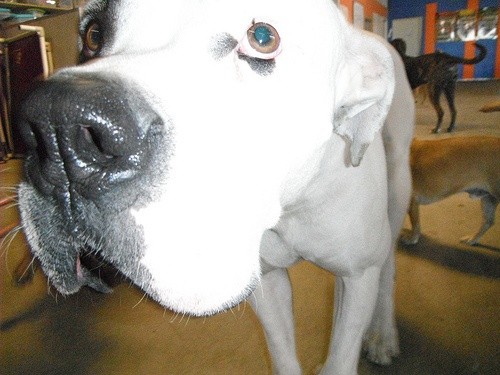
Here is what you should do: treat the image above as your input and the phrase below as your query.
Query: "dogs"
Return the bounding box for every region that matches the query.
[388,38,487,133]
[0,0,414,375]
[401,136,500,245]
